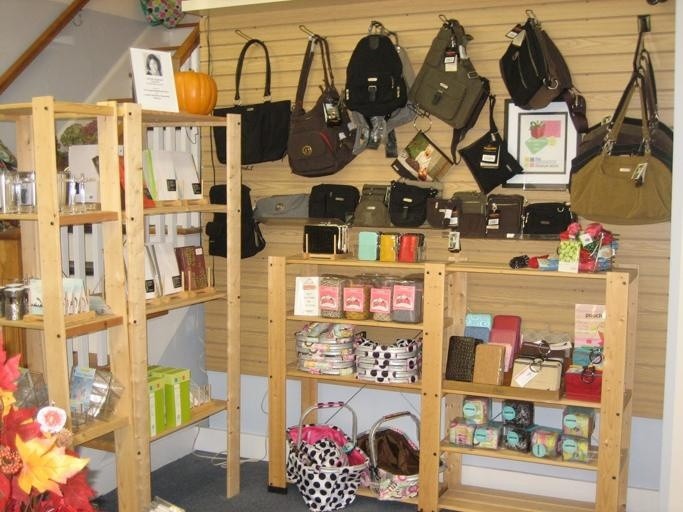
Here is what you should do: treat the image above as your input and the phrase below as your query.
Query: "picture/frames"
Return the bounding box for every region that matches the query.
[501,97,582,192]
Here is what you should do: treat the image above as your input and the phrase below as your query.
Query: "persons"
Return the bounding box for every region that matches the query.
[143,53,163,77]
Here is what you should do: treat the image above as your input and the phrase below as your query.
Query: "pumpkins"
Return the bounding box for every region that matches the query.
[175,67,218,115]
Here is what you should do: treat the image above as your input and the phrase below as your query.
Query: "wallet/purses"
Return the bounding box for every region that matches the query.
[145,241,210,301]
[465,312,492,329]
[464,325,490,344]
[493,314,521,331]
[357,231,426,262]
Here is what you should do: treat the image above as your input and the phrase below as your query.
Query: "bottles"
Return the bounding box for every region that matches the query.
[0,169,37,213]
[0,274,29,321]
[319,272,424,323]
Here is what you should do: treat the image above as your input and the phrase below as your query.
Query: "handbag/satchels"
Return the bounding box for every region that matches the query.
[206,184,266,259]
[285,35,353,178]
[213,39,291,166]
[340,21,416,144]
[489,191,524,241]
[384,181,439,227]
[301,184,360,252]
[510,356,562,392]
[406,14,492,131]
[453,191,487,238]
[565,368,602,401]
[571,345,604,366]
[457,96,524,195]
[473,345,505,384]
[445,335,483,382]
[354,183,389,227]
[389,130,454,186]
[522,341,573,362]
[499,10,590,132]
[523,201,572,238]
[490,329,519,373]
[568,50,674,226]
[428,194,459,227]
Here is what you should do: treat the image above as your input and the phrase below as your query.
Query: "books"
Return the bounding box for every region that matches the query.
[68,365,95,415]
[120,243,208,300]
[82,370,112,419]
[90,154,156,212]
[141,149,201,201]
